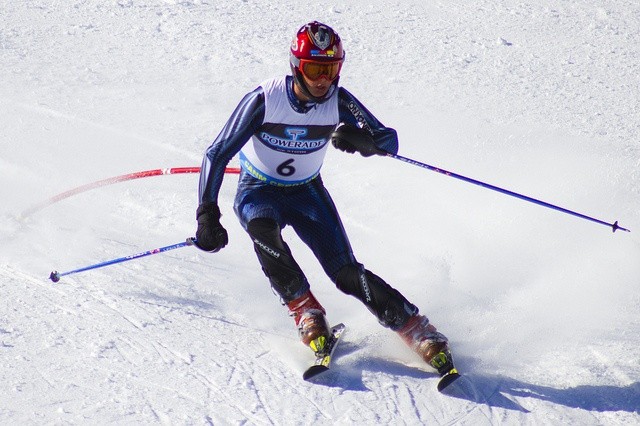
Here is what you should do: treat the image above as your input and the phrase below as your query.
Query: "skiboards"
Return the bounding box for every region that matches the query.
[301,323,461,392]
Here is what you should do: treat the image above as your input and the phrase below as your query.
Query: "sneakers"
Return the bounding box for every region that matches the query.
[396,315,449,362]
[286,290,329,346]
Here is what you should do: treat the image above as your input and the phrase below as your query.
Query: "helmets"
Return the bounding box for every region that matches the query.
[290,21,345,103]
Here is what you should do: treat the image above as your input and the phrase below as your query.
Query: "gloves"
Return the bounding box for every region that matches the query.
[195,203,228,252]
[331,124,375,157]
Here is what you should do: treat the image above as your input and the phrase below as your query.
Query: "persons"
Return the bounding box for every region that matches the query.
[192,19,450,370]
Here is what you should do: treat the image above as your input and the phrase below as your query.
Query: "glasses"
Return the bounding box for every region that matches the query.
[290,50,345,81]
